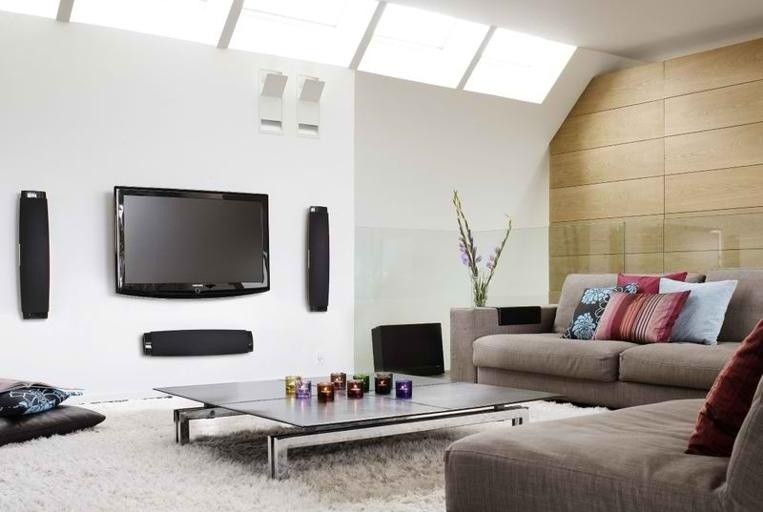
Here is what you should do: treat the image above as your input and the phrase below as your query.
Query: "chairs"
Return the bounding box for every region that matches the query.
[437,376,762,512]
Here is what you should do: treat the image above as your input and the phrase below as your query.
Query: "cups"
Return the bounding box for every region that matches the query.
[285,372,413,401]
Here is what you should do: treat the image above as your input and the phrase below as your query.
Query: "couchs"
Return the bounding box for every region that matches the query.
[447,268,762,410]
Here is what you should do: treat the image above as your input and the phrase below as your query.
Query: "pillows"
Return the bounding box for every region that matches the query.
[614,268,689,293]
[0,403,107,446]
[589,286,690,345]
[0,386,86,420]
[556,280,641,340]
[680,314,761,456]
[656,275,739,348]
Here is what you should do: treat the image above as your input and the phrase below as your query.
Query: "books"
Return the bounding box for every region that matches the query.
[0,378,63,393]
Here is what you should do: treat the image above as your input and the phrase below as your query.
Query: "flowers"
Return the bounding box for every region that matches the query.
[450,186,513,307]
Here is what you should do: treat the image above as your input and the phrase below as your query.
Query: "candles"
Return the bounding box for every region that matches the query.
[278,369,415,403]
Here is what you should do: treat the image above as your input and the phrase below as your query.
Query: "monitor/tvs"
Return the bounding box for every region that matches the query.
[112,185,270,300]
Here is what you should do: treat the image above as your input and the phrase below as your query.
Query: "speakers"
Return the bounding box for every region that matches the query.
[142,329,254,356]
[307,206,330,312]
[18,190,49,319]
